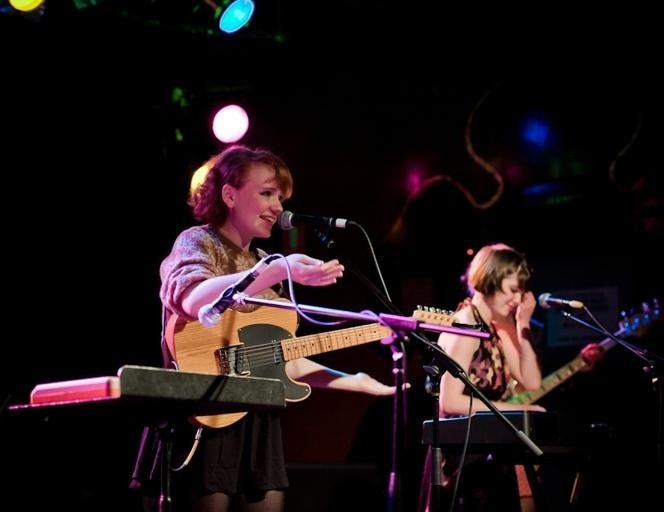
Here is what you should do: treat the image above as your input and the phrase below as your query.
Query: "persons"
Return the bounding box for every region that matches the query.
[127,143,412,510]
[418,239,547,512]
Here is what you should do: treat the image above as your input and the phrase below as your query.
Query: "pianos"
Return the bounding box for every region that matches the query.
[420,404,662,461]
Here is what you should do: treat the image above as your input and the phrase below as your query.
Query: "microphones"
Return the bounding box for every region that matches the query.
[538,293,584,310]
[197,256,272,328]
[277,210,357,231]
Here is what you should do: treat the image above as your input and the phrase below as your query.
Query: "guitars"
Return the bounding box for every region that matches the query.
[166,281,486,432]
[438,296,664,490]
